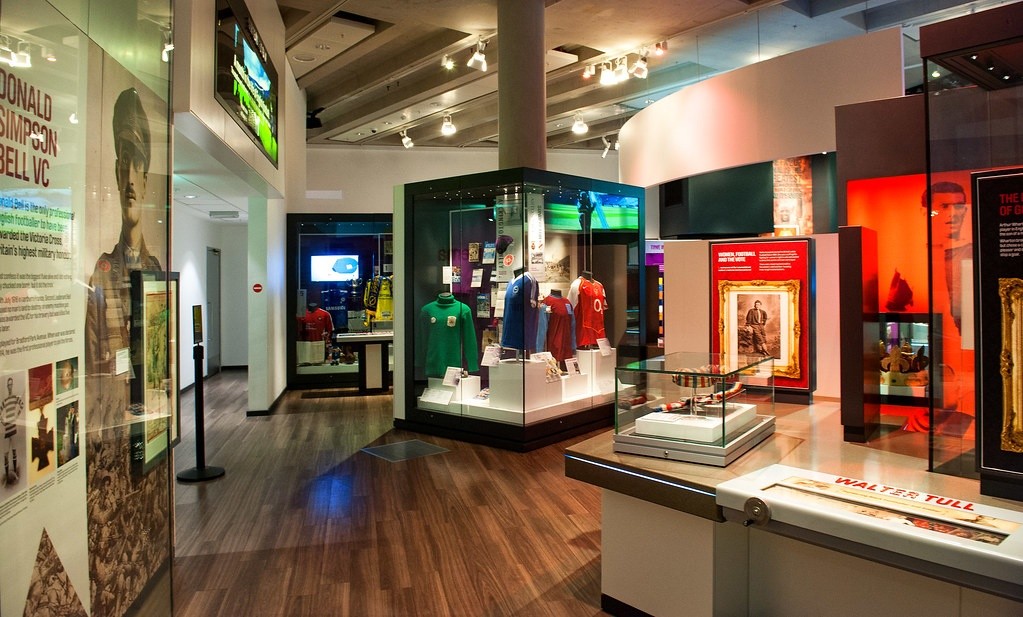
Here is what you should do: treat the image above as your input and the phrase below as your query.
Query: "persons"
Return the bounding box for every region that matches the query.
[746,300,771,355]
[501,266,538,350]
[84,86,162,435]
[0,377,24,479]
[297,303,335,358]
[885,182,973,312]
[415,292,479,378]
[566,271,608,346]
[536,289,577,361]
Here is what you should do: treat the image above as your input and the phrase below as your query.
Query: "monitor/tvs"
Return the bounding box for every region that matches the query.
[311,255,359,281]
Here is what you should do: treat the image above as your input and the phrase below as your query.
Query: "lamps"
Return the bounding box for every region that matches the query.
[572,112,588,135]
[400,130,414,149]
[584,42,664,85]
[442,114,456,135]
[467,38,490,72]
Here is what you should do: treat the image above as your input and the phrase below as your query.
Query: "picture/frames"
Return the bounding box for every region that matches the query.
[709,237,817,393]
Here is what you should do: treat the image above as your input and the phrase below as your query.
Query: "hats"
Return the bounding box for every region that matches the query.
[496,235,514,254]
[113,87,151,170]
